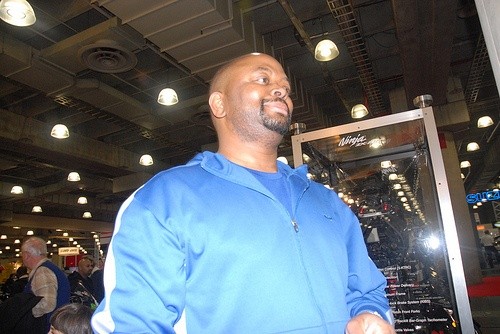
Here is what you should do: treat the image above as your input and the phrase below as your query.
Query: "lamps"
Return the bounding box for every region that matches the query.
[50,106,69,139]
[277,100,500,244]
[314,17,339,62]
[0,156,104,258]
[139,139,153,166]
[0,0,36,27]
[157,67,179,106]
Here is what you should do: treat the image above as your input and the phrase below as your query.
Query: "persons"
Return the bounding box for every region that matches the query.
[480,230,500,268]
[0,236,104,334]
[90,52,396,334]
